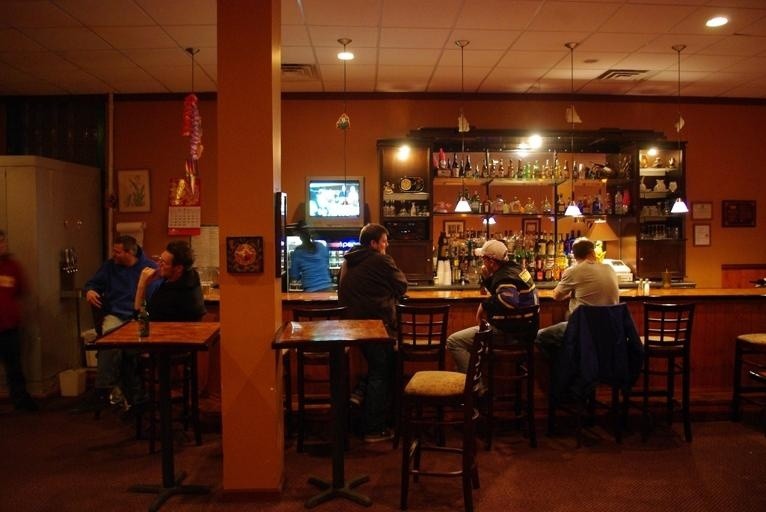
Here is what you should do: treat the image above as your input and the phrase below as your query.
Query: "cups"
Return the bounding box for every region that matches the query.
[201,284,211,297]
[661,272,673,289]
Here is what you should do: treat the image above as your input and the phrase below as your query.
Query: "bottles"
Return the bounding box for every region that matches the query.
[640,178,667,194]
[384,201,428,216]
[328,251,348,282]
[642,225,680,241]
[137,299,150,338]
[431,146,633,283]
[643,201,671,217]
[638,278,650,292]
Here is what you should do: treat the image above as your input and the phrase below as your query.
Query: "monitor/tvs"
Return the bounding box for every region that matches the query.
[305,175,365,228]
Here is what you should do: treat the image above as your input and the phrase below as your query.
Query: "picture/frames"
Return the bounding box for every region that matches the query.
[693,221,711,247]
[115,169,152,214]
[690,200,714,220]
[223,234,267,275]
[444,220,465,239]
[720,198,757,228]
[522,217,540,236]
[274,189,286,280]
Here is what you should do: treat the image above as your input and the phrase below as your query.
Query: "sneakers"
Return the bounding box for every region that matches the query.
[351,382,364,406]
[365,427,395,443]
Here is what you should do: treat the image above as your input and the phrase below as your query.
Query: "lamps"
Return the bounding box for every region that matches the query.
[561,40,583,217]
[452,39,474,215]
[483,215,496,225]
[669,43,691,217]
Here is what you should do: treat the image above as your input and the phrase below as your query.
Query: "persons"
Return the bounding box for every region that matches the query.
[0,230,34,410]
[536,240,620,362]
[309,184,360,217]
[446,239,540,400]
[106,239,207,417]
[336,222,408,443]
[288,232,334,293]
[81,235,158,405]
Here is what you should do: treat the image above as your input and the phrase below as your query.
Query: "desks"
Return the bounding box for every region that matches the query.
[83,317,221,511]
[271,317,397,512]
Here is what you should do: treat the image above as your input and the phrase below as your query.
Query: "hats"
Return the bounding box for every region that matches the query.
[474,239,509,261]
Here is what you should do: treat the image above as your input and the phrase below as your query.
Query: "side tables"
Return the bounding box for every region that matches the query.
[200,283,765,405]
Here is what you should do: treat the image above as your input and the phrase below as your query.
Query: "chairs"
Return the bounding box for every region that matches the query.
[546,300,633,446]
[286,303,453,454]
[628,301,697,444]
[401,319,498,510]
[730,333,765,430]
[74,291,201,458]
[476,303,542,451]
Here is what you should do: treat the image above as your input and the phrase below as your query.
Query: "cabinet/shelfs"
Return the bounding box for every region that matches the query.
[370,121,688,289]
[0,149,104,405]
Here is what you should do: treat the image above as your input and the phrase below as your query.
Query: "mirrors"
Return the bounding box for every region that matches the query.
[432,148,625,269]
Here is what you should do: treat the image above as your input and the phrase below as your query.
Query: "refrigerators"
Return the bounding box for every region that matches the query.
[284,225,364,291]
